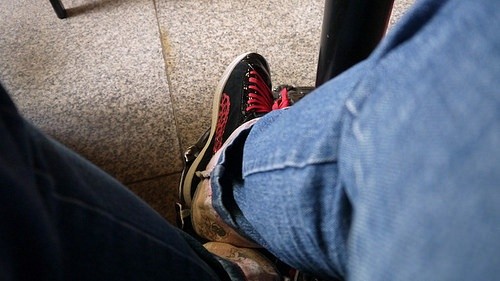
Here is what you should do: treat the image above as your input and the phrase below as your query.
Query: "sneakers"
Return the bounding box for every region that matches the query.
[183,52,290,208]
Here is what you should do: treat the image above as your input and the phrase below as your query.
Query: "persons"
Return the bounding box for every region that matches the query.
[0,0,500,281]
